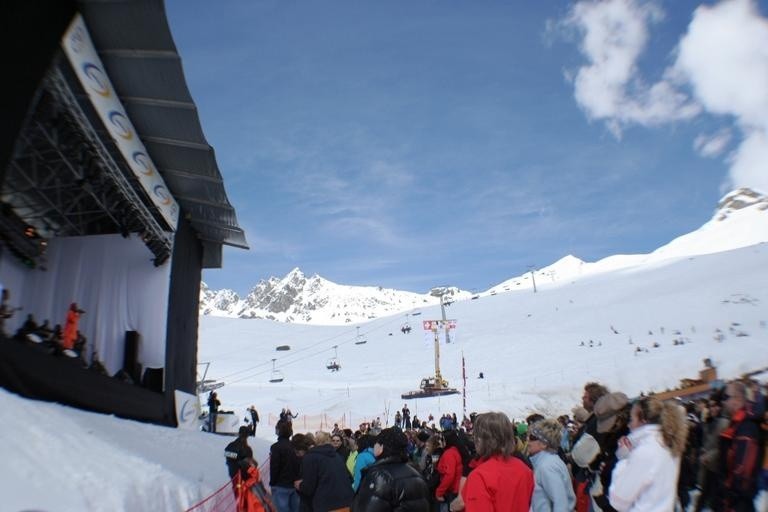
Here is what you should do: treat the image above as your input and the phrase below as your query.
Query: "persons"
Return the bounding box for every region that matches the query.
[512,380,689,512]
[1,289,15,334]
[24,302,108,376]
[225,404,535,512]
[401,325,408,333]
[676,357,768,512]
[202,390,221,434]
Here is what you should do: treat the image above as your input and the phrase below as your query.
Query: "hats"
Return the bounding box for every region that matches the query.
[594,392,629,434]
[368,428,409,449]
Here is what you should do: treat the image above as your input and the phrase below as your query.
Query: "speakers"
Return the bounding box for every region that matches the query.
[143,367,164,393]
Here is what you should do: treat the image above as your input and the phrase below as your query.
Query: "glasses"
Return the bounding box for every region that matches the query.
[529,434,539,442]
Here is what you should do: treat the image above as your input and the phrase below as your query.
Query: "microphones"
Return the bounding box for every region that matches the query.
[76,308,86,314]
